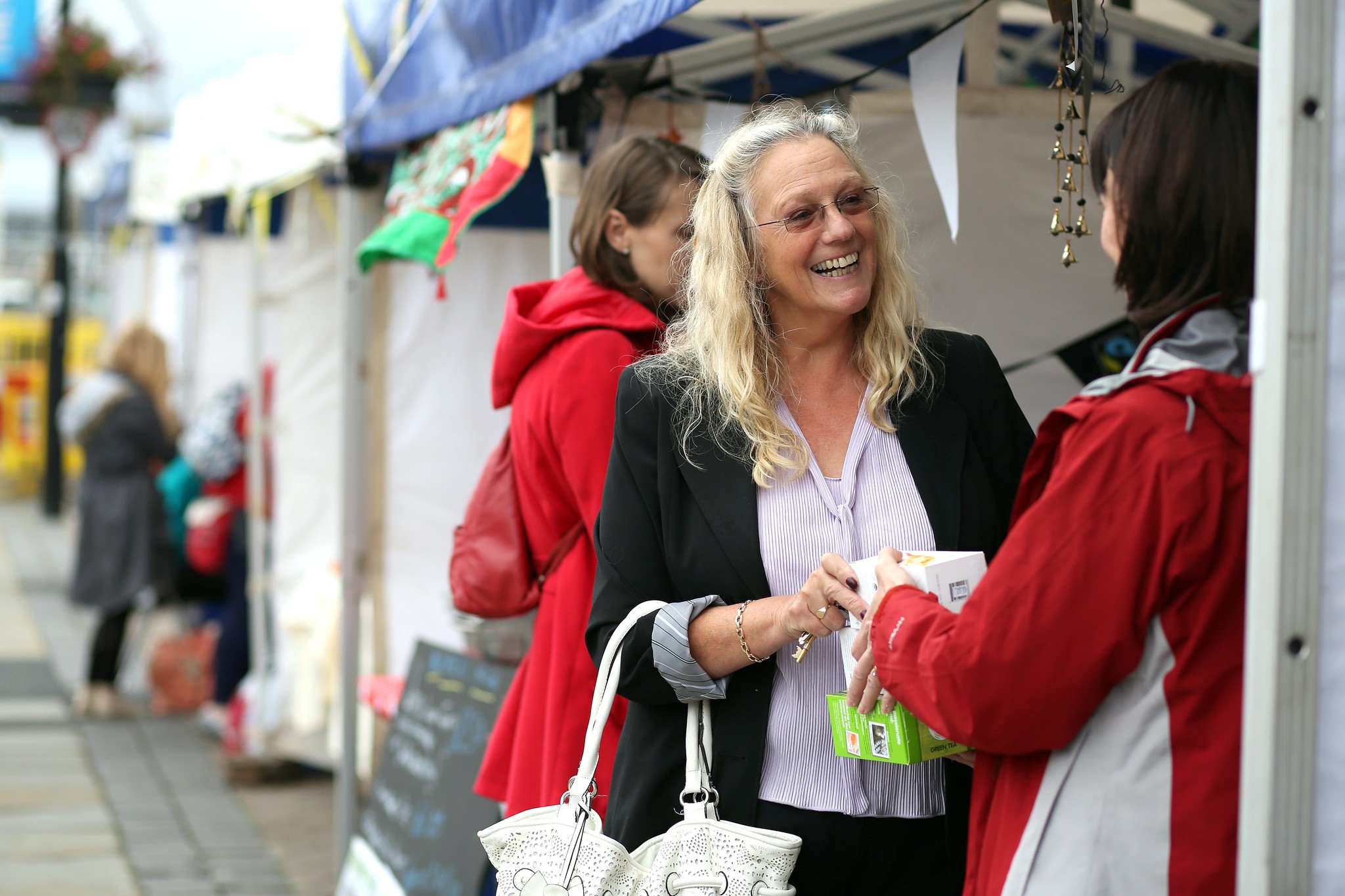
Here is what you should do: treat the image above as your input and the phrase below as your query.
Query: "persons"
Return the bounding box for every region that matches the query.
[54,326,187,717]
[193,359,276,741]
[581,97,1037,895]
[845,57,1260,896]
[472,133,716,896]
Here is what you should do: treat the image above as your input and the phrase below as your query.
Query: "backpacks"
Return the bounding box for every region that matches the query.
[450,420,587,622]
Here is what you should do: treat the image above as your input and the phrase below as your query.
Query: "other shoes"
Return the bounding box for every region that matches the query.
[72,682,236,742]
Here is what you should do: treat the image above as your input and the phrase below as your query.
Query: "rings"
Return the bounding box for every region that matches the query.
[872,664,878,677]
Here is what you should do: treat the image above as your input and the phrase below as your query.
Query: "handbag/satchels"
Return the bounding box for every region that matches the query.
[476,600,804,896]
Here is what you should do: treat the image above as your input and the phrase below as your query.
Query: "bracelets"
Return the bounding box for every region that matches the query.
[735,600,770,663]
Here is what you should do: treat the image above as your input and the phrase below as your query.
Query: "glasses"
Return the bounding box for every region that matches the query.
[744,184,879,233]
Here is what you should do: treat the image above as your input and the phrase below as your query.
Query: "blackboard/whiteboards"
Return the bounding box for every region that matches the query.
[355,640,523,894]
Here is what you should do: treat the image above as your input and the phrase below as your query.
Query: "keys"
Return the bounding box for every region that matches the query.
[791,600,849,664]
